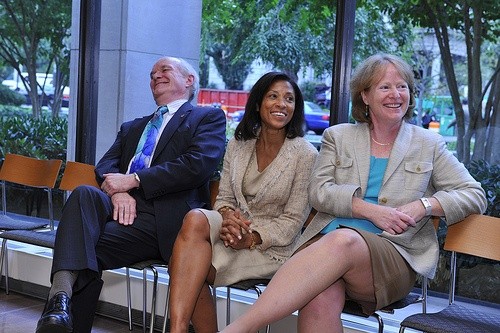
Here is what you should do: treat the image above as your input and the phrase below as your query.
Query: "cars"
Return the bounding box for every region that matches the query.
[404,95,470,137]
[10,82,70,107]
[227,100,330,135]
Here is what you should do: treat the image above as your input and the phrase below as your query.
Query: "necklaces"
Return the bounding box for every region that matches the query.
[371,136,393,146]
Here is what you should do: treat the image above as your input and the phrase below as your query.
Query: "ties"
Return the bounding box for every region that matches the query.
[129,105,169,175]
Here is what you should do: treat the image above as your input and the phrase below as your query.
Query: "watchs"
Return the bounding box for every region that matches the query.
[134,172,140,185]
[419,197,432,217]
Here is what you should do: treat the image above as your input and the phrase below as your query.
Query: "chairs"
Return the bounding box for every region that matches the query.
[0,153,98,294]
[397,213,500,333]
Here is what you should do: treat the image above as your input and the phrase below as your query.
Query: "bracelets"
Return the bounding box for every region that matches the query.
[249,233,256,250]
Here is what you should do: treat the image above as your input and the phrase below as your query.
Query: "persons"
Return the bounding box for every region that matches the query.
[216,53,488,333]
[168,71,320,333]
[34,56,226,333]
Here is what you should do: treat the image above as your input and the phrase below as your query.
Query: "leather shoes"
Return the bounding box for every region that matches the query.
[36,288,72,333]
